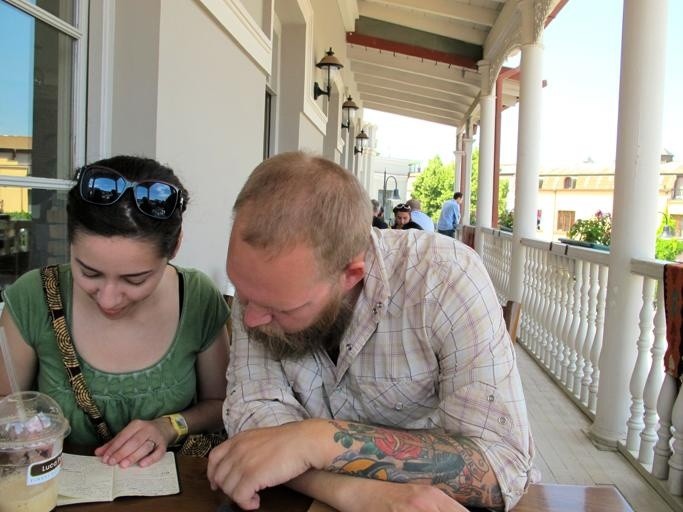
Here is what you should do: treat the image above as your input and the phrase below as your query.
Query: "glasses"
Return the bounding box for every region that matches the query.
[396,203,411,210]
[74,164,184,220]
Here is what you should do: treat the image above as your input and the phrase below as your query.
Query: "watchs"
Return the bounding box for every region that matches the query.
[162,413,189,444]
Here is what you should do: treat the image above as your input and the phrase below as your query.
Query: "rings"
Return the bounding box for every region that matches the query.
[146,438,157,449]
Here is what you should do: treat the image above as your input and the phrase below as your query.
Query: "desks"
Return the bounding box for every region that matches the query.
[0,446,636,512]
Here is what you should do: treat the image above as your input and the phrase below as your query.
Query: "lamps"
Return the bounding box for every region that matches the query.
[311,46,369,154]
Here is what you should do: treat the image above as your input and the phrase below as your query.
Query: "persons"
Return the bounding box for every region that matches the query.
[371,198,435,231]
[437,192,464,238]
[205,150,541,512]
[0,154,231,468]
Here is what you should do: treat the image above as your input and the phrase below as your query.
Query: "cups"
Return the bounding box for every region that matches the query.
[0,391,71,512]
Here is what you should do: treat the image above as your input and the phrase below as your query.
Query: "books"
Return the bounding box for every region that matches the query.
[57,451,183,506]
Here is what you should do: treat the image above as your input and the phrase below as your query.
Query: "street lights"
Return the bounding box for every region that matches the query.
[656,209,673,242]
[382,168,400,219]
